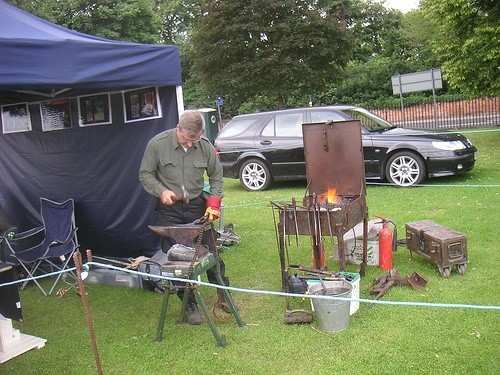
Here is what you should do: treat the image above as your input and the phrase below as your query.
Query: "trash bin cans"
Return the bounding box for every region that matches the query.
[197,107,219,146]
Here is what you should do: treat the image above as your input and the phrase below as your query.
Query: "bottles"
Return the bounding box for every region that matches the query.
[292,273,300,282]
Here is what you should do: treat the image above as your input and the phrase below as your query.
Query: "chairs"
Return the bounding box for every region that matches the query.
[4,197,83,296]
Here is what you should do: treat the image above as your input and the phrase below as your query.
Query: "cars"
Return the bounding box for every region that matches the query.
[214,105,478,191]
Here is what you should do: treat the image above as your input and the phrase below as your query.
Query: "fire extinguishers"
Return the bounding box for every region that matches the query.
[373,214,397,270]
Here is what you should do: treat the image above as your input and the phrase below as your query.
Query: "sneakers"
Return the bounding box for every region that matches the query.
[185,302,205,324]
[218,277,229,308]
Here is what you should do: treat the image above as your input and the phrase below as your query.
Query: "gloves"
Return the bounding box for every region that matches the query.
[204,197,221,223]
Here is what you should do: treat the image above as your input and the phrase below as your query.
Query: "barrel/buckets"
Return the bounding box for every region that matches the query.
[309,281,353,332]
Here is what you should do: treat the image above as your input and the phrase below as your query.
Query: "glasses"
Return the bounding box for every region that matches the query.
[180,131,201,143]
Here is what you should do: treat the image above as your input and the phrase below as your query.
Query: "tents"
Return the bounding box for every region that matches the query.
[0,0,185,272]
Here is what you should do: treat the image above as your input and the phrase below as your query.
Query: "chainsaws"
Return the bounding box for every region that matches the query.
[360,275,408,311]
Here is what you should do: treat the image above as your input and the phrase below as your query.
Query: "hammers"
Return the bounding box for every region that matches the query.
[297,266,346,280]
[172,186,189,203]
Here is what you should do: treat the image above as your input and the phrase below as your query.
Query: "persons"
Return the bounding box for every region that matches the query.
[139,109,239,325]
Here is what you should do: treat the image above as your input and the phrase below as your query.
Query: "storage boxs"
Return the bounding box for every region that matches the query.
[307,273,362,316]
[342,233,381,267]
[406,220,469,276]
